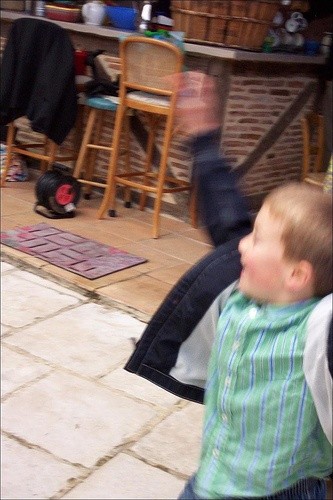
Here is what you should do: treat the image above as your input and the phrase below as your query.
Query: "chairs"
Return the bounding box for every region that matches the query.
[96,38,198,240]
[300,114,327,187]
[0,17,84,186]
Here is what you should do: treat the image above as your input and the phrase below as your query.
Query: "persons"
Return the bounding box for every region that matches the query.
[123,71,333,500]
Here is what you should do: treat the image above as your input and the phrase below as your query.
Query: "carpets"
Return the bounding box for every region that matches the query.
[0,222,146,280]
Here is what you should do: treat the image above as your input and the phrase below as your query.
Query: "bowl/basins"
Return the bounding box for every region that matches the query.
[105,6,138,27]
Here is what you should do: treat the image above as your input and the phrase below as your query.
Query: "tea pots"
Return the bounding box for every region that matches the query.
[82,1,106,26]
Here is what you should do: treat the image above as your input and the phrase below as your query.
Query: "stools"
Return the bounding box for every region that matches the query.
[73,96,158,217]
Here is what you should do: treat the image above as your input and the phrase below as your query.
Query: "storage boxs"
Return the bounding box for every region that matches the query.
[170,0,279,50]
